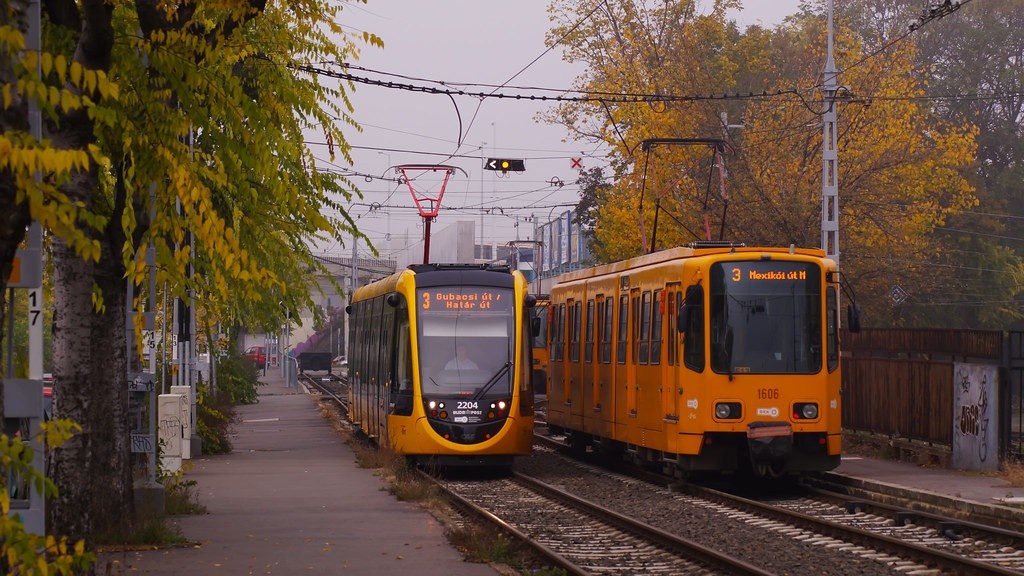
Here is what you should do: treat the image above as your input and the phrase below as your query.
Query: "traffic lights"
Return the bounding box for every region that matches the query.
[484,157,526,172]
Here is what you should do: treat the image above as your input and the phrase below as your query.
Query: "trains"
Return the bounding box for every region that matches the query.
[526,238,864,487]
[348,252,544,469]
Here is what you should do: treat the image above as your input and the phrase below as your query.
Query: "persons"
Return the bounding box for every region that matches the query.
[445,344,478,370]
[728,325,766,373]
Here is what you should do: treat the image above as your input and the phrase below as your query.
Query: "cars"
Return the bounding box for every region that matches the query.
[242,345,277,369]
